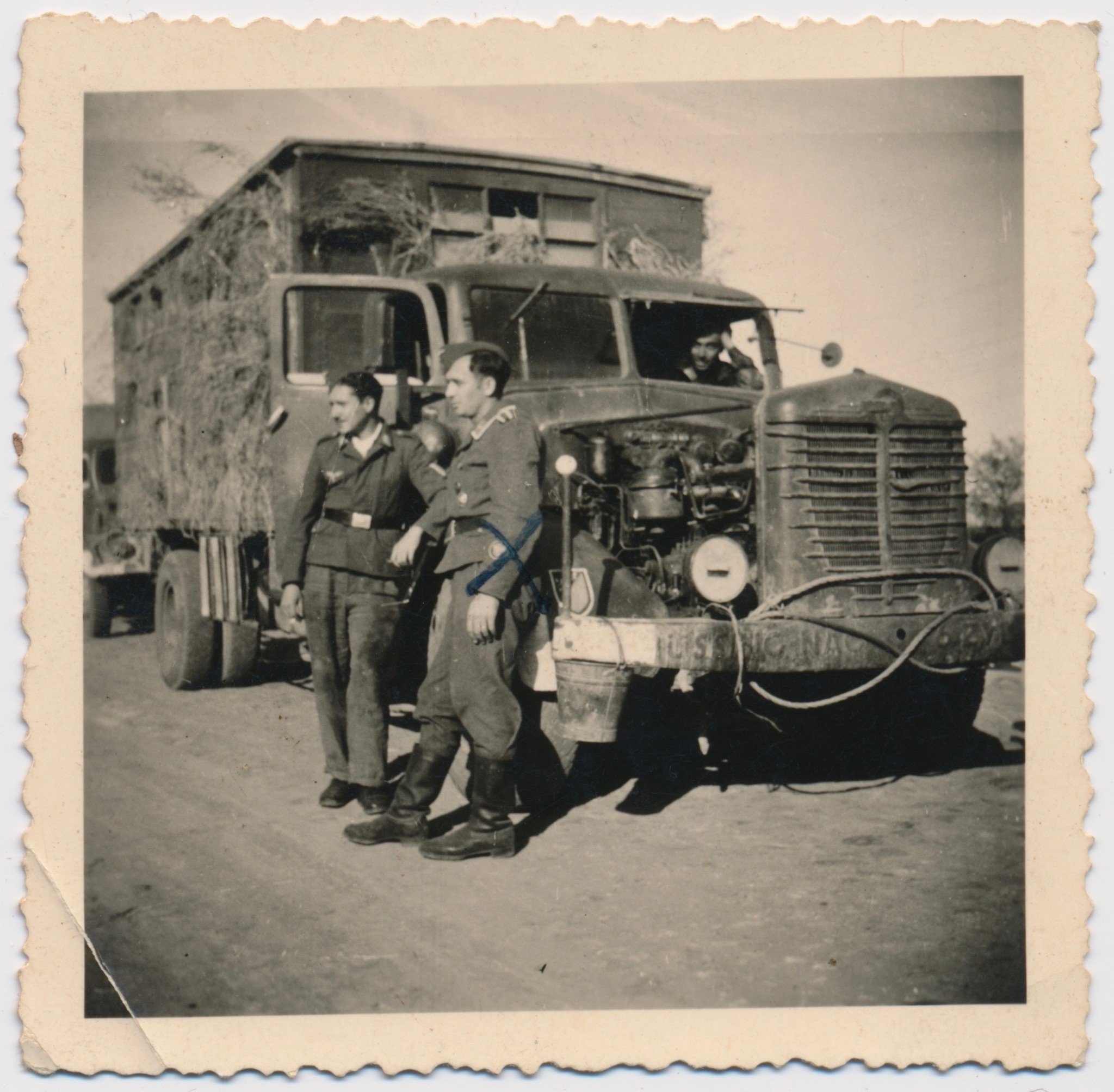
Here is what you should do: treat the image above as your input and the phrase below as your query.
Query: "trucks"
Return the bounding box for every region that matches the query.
[83,402,158,639]
[108,135,1024,791]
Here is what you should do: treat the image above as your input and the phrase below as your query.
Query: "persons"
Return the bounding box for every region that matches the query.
[672,309,766,391]
[278,370,454,815]
[343,343,543,863]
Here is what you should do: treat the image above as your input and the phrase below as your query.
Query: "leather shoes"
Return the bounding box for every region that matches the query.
[319,779,352,809]
[358,783,393,813]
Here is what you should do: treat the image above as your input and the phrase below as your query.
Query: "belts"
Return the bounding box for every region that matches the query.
[442,518,483,543]
[322,507,399,530]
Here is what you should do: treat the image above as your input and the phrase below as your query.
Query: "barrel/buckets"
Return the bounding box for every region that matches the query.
[555,615,634,744]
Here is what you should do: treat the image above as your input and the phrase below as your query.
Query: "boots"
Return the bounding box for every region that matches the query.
[344,742,452,844]
[420,755,518,861]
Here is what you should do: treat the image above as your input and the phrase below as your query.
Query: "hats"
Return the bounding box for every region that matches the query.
[437,339,512,366]
